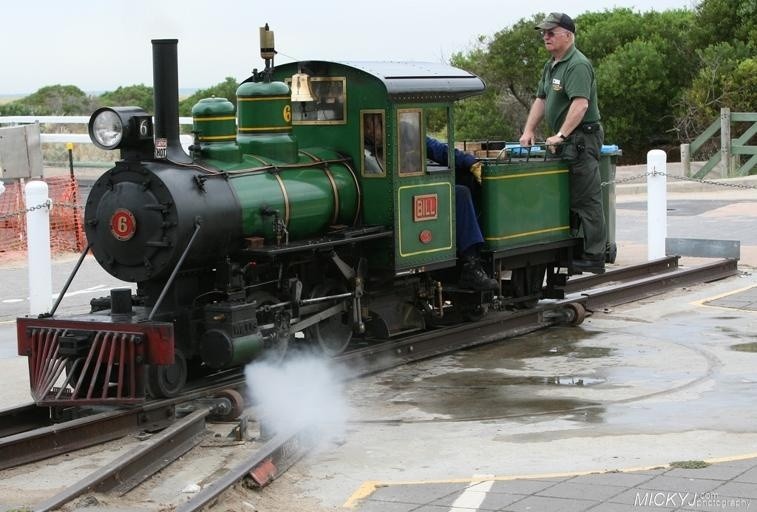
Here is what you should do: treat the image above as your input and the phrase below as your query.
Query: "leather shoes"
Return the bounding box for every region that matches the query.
[571,258,605,274]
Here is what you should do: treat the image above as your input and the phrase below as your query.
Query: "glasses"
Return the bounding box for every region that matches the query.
[540,30,570,37]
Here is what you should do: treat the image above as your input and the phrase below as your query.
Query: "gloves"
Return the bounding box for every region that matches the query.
[470,160,486,183]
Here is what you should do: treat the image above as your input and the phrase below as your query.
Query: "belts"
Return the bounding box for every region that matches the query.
[573,119,601,132]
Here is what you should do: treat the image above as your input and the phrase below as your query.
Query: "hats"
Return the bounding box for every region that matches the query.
[534,12,575,33]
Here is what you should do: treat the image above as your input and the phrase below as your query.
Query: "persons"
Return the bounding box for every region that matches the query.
[515,11,608,277]
[364,115,499,290]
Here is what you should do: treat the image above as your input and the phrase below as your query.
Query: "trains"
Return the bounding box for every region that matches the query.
[16,22,623,408]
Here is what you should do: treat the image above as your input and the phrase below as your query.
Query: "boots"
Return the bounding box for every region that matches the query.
[458,263,499,290]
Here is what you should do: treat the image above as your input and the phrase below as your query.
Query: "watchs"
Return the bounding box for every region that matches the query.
[556,132,565,140]
